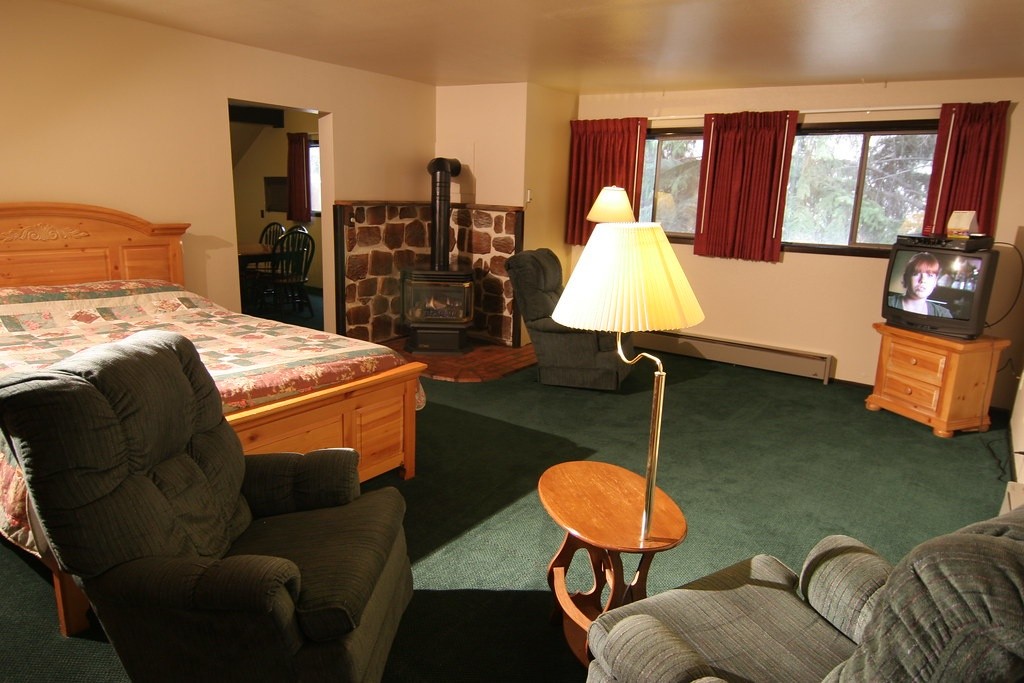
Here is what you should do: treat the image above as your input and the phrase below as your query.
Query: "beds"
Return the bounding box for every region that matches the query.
[0,178,429,643]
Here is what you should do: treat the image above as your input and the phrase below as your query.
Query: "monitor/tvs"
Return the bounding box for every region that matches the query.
[881,243,999,341]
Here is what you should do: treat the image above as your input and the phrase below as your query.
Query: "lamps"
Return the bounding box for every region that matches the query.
[587,186,637,225]
[551,221,709,535]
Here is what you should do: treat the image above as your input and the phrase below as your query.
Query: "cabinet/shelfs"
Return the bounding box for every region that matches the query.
[867,321,1011,440]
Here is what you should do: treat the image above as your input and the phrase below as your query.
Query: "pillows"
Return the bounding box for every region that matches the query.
[0,275,210,296]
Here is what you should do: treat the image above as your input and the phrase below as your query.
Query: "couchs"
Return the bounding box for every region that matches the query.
[506,248,637,390]
[1,333,415,683]
[587,507,1024,683]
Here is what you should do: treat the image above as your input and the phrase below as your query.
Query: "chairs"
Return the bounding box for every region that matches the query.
[244,220,316,318]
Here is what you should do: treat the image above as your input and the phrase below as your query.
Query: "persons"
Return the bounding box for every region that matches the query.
[888,252,953,318]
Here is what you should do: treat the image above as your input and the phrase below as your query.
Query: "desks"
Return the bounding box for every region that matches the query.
[539,460,689,666]
[238,242,307,292]
